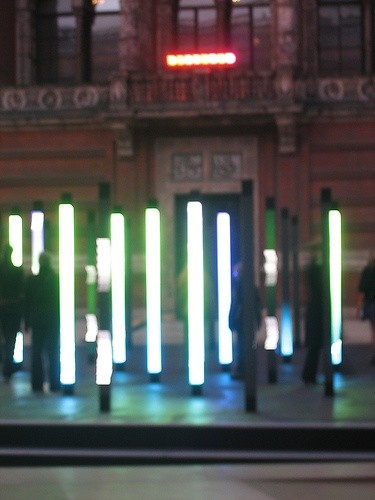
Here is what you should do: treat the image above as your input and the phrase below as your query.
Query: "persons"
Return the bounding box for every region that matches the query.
[229,262,265,379]
[1,245,24,377]
[25,252,59,392]
[356,250,374,333]
[299,241,330,385]
